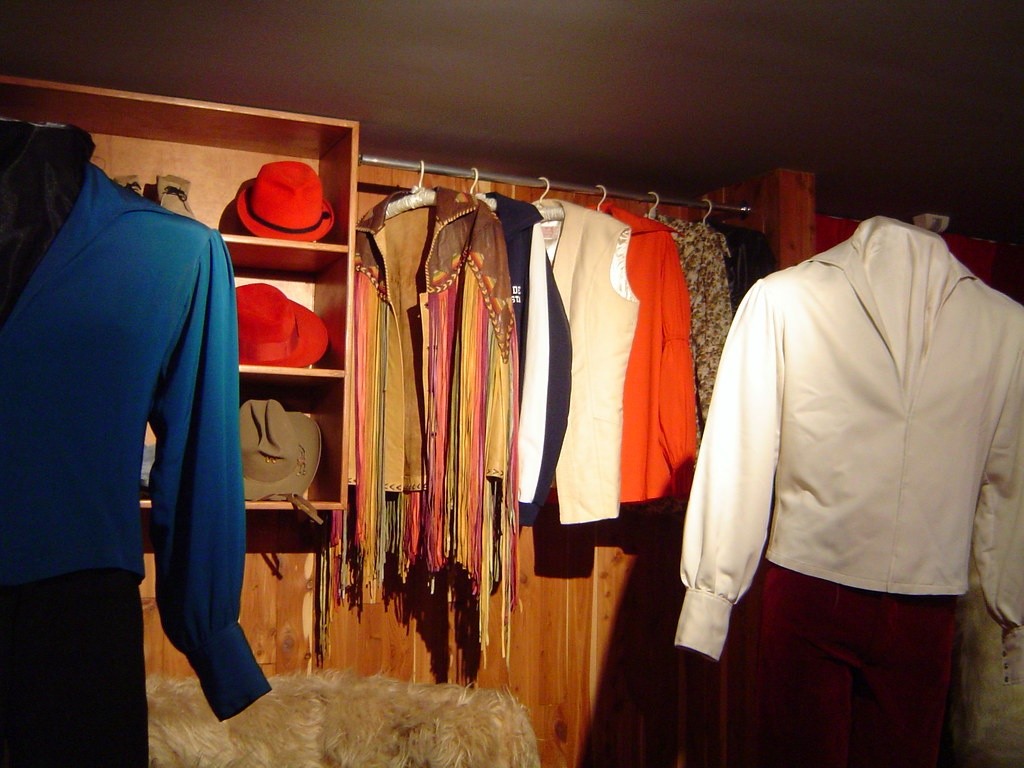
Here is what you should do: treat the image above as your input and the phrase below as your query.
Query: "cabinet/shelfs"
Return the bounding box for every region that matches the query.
[1,73,361,511]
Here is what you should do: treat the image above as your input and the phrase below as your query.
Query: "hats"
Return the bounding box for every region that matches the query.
[235,282,331,368]
[236,158,335,240]
[235,398,323,502]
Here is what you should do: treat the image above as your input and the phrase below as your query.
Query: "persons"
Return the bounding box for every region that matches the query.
[0,127,273,768]
[672,216,1023,768]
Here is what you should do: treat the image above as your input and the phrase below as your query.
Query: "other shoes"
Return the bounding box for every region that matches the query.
[115,174,143,198]
[154,173,199,222]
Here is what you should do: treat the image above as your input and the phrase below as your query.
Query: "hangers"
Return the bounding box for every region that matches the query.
[374,160,724,240]
